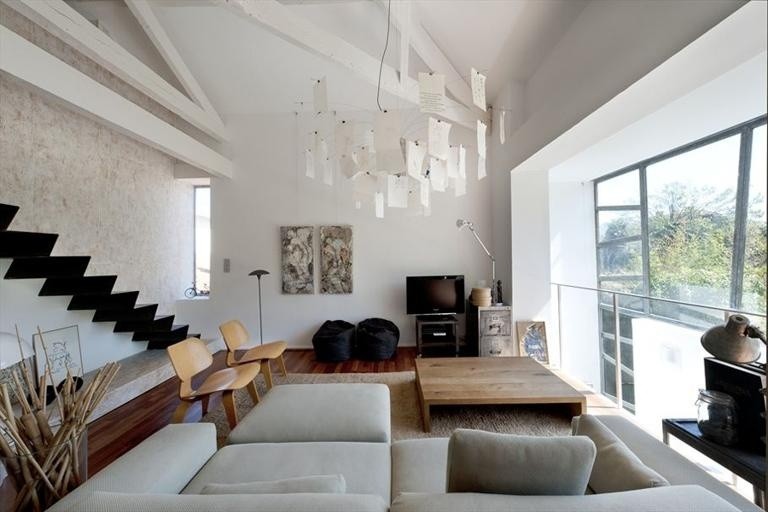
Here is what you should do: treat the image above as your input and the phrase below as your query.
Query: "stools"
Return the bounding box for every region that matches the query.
[228,384,390,440]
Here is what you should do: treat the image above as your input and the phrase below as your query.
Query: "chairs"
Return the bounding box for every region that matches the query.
[168,338,259,431]
[220,321,288,389]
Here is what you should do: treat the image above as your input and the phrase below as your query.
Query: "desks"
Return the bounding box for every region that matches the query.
[661,417,767,508]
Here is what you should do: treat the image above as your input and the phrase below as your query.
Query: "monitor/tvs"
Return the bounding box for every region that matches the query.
[406,274,465,315]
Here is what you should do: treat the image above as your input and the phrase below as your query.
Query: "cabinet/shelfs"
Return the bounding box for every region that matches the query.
[417,315,460,358]
[466,300,514,358]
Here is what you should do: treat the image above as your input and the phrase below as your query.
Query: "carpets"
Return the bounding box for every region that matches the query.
[202,372,579,437]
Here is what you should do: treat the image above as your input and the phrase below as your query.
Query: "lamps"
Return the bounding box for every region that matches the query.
[456,220,496,303]
[700,317,767,366]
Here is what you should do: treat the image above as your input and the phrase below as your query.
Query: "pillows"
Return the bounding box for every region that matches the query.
[200,474,346,493]
[444,428,595,496]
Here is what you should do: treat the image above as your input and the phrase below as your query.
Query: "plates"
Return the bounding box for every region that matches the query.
[25,385,59,408]
[54,375,84,395]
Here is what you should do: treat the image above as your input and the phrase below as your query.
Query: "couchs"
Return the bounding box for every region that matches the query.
[44,414,766,512]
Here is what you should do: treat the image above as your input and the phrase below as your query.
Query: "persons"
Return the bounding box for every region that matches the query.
[481,322,503,357]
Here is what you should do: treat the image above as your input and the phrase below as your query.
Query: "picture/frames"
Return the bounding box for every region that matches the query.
[33,324,85,393]
